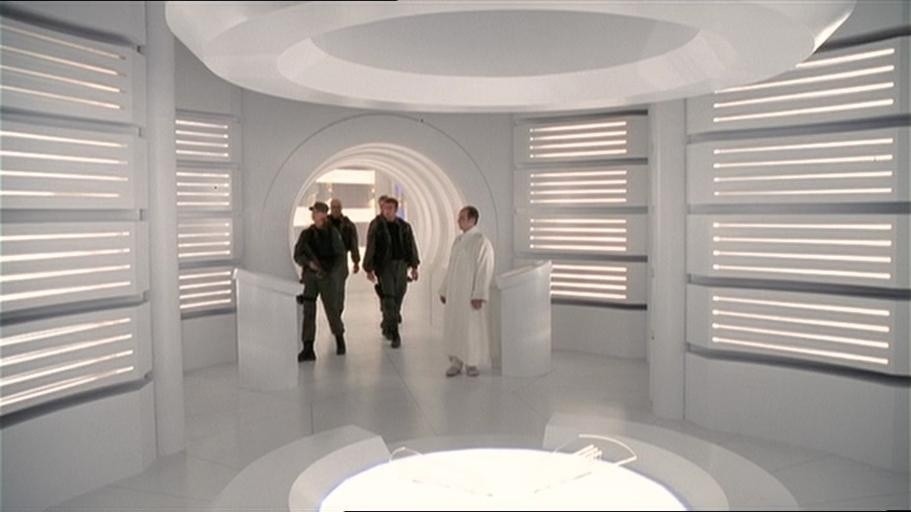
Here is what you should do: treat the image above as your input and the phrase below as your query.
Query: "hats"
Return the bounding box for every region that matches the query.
[309,202,329,213]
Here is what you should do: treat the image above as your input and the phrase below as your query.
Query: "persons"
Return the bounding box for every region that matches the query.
[293,202,349,361]
[367,195,403,322]
[438,206,493,376]
[362,197,420,348]
[328,198,360,273]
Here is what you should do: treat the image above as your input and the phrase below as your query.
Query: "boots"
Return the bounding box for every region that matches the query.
[298,339,316,362]
[336,331,346,355]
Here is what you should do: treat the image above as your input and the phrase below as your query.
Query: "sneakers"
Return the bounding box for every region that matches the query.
[466,364,480,377]
[381,321,401,348]
[446,360,463,376]
